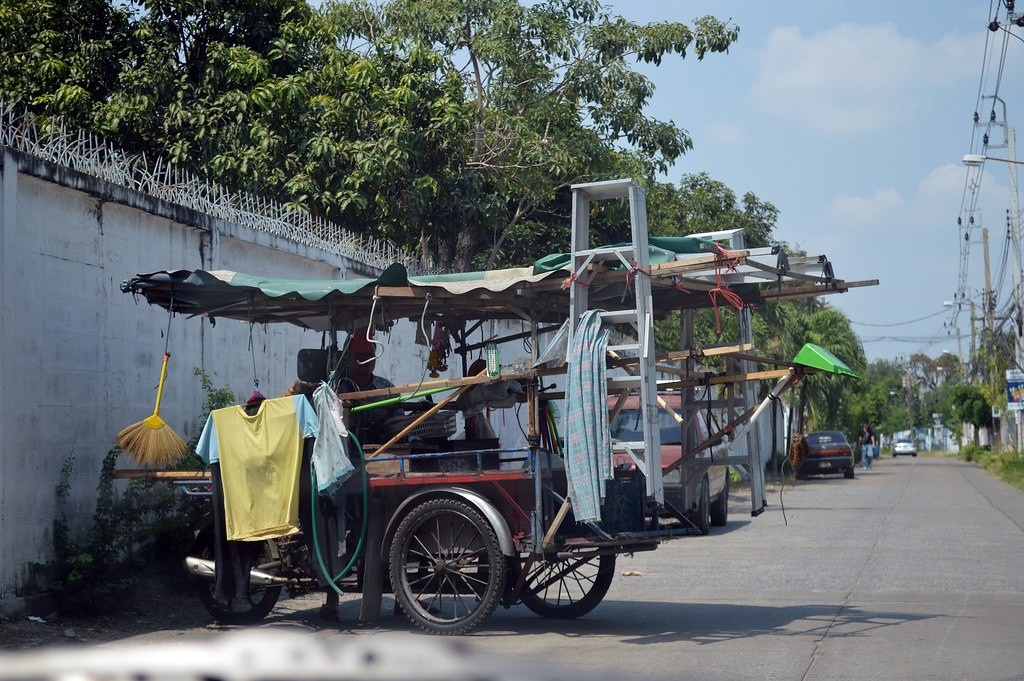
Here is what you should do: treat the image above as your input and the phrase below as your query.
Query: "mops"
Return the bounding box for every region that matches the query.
[364,341,500,461]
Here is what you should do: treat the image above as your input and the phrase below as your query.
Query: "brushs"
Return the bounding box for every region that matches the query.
[788,377,810,468]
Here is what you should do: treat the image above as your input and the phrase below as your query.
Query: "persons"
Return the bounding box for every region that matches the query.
[858,422,875,470]
[318,330,440,622]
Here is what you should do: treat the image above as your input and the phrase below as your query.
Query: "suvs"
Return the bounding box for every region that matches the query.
[607,389,731,533]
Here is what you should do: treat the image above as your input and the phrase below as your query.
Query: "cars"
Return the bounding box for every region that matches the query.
[892,439,918,458]
[793,430,855,481]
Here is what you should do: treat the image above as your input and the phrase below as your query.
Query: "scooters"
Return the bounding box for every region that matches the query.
[173,446,702,636]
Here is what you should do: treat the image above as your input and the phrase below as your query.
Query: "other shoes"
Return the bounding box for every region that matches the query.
[394,595,436,616]
[320,602,340,617]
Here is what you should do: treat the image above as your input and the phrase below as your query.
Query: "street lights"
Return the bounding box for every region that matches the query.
[889,391,914,444]
[943,301,1002,462]
[942,349,971,443]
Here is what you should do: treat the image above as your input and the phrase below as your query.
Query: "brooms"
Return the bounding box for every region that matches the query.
[117,353,192,471]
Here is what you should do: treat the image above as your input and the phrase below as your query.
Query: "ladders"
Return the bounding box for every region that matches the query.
[682,227,767,517]
[568,176,664,512]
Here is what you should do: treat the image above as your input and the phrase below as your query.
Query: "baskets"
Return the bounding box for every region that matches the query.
[382,410,458,443]
